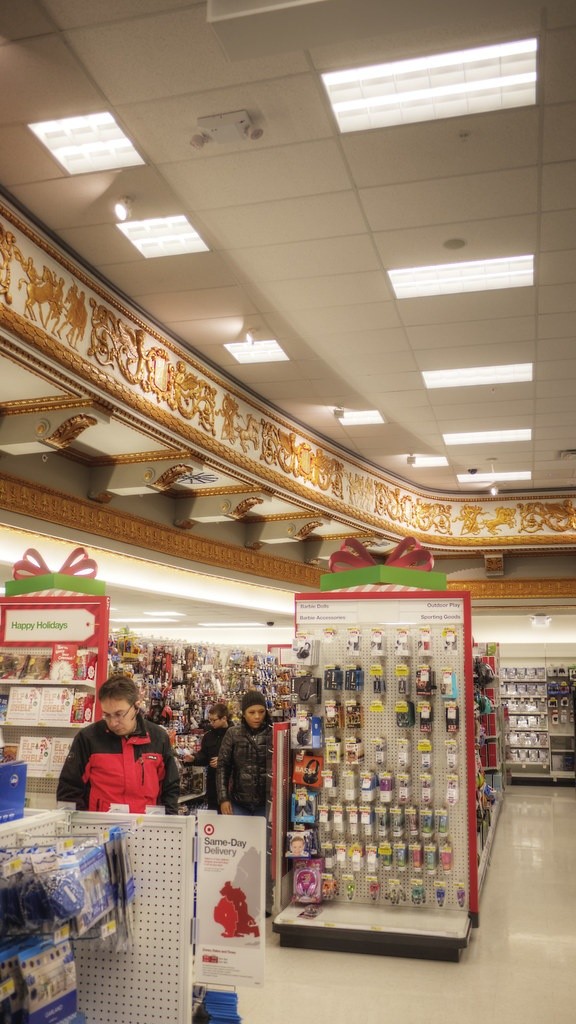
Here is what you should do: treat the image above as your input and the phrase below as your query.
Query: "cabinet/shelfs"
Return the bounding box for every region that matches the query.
[475,640,506,896]
[0,595,111,811]
[499,667,576,786]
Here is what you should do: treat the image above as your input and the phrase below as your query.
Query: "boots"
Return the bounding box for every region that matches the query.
[266,882,274,917]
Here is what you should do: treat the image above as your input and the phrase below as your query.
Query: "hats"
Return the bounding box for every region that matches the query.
[241,691,267,712]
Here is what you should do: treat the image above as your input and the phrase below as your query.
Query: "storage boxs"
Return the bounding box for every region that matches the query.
[284,640,326,903]
[6,643,98,773]
[476,656,504,803]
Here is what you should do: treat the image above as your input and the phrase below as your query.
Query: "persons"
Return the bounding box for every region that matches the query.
[56,674,180,816]
[184,703,234,815]
[215,691,275,816]
[286,835,310,858]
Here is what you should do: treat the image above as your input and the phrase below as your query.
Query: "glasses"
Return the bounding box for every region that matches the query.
[208,717,221,724]
[101,703,134,720]
